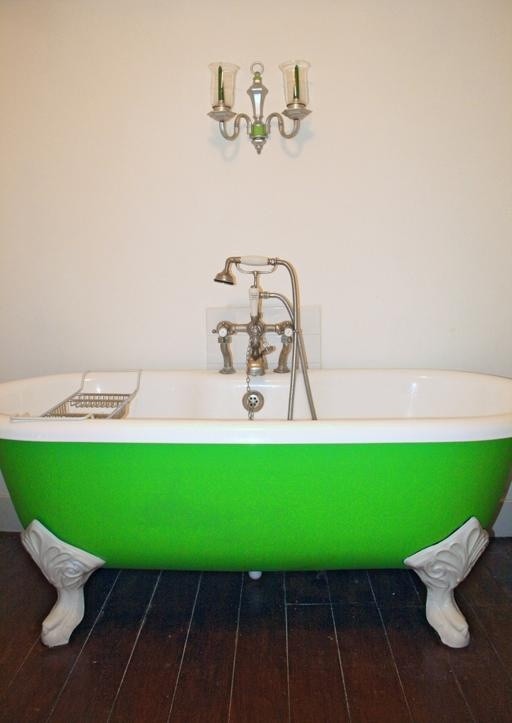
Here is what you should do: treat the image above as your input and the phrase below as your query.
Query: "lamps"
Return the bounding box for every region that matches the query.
[206,55,312,151]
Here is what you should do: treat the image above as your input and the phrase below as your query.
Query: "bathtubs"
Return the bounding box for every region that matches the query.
[0,366,510,571]
